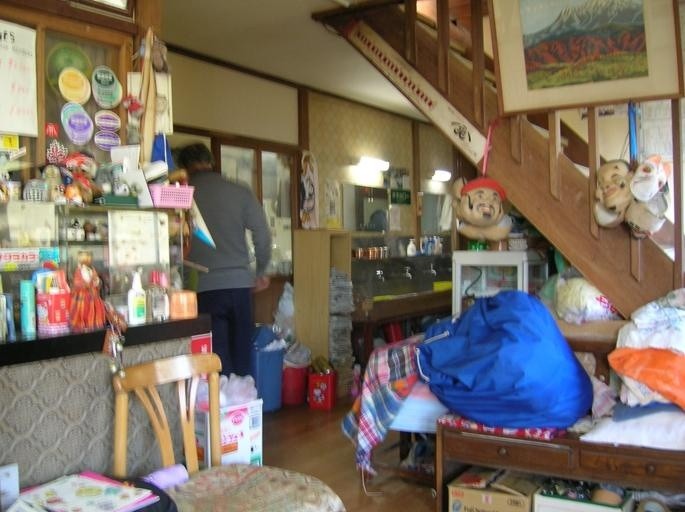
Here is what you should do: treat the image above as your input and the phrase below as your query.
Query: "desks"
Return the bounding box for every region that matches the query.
[341,333,452,488]
[0,472,177,512]
[351,291,452,366]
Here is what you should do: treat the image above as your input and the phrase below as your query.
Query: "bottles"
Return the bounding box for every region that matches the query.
[425,236,442,255]
[71,217,94,241]
[126,272,168,327]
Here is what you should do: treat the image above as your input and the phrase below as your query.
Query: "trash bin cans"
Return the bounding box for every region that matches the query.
[249,325,285,415]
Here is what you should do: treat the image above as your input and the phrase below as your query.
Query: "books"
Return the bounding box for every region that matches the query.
[18,470,160,512]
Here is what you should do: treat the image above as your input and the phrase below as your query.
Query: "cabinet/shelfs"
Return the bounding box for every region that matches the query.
[292,228,351,399]
[0,1,135,201]
[436,415,685,512]
[452,249,550,324]
[0,201,185,302]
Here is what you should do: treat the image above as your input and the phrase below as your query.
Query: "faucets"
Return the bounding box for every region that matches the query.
[430,263,438,276]
[376,270,385,283]
[404,266,413,280]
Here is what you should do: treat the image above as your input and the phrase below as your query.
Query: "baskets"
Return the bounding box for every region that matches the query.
[147,185,195,209]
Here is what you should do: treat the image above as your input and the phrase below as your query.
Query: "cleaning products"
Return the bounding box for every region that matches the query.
[20,280,37,336]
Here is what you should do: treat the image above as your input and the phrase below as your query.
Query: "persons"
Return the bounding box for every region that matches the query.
[175,141,272,375]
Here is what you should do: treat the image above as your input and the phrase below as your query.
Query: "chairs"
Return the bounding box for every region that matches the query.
[111,351,347,512]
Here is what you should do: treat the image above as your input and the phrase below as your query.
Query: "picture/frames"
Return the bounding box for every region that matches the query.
[487,0,685,118]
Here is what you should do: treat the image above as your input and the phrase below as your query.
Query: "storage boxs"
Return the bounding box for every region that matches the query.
[446,468,634,512]
[186,400,263,469]
